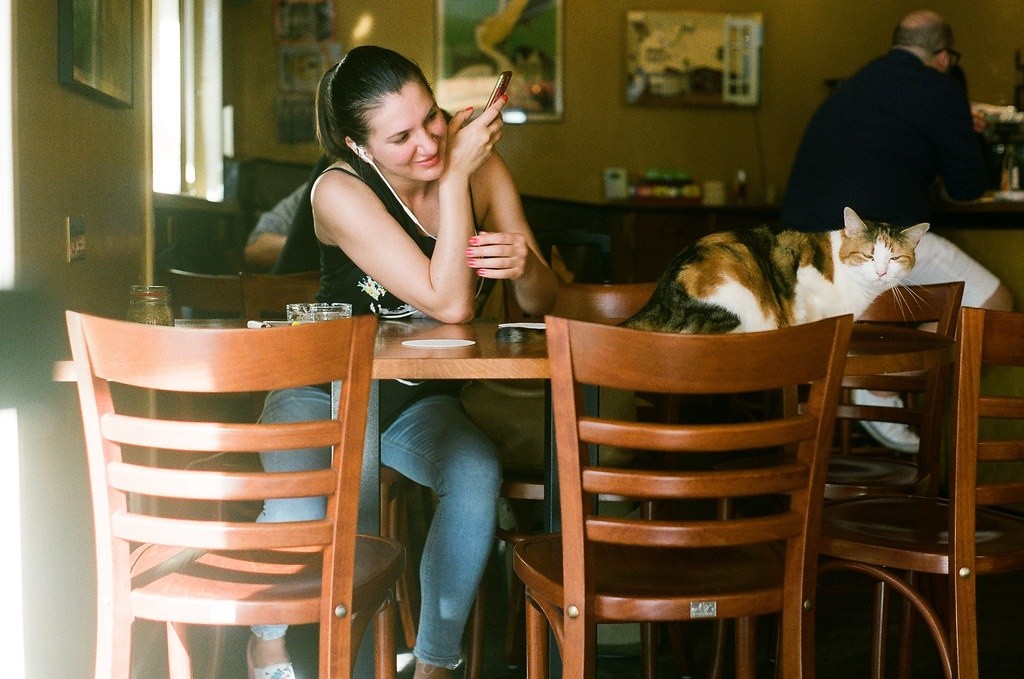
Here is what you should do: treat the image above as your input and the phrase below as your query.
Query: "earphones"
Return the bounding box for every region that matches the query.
[352,143,373,165]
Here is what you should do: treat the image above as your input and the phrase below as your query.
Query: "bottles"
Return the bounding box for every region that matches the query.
[127,285,174,326]
[636,169,702,203]
[1000,145,1022,192]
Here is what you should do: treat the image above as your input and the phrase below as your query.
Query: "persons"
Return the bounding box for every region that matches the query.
[245,44,558,678]
[239,181,309,272]
[778,11,1012,452]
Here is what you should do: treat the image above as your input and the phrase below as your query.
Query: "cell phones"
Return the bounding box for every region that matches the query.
[483,70,513,111]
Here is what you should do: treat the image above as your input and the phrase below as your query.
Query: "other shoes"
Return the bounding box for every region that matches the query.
[246,634,295,679]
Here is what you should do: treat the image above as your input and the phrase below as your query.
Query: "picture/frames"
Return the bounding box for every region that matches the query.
[624,11,762,105]
[435,0,562,124]
[57,0,134,109]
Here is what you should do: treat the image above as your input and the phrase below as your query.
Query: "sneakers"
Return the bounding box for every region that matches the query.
[852,388,921,452]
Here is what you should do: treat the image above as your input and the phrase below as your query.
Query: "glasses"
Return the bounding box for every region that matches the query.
[933,47,962,69]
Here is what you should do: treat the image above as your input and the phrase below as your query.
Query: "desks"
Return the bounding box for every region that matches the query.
[45,322,958,678]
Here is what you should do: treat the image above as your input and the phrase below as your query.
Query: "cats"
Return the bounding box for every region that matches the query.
[612,205,931,332]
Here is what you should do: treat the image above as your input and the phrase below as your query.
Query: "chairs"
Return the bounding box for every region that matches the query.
[68,270,1024,679]
